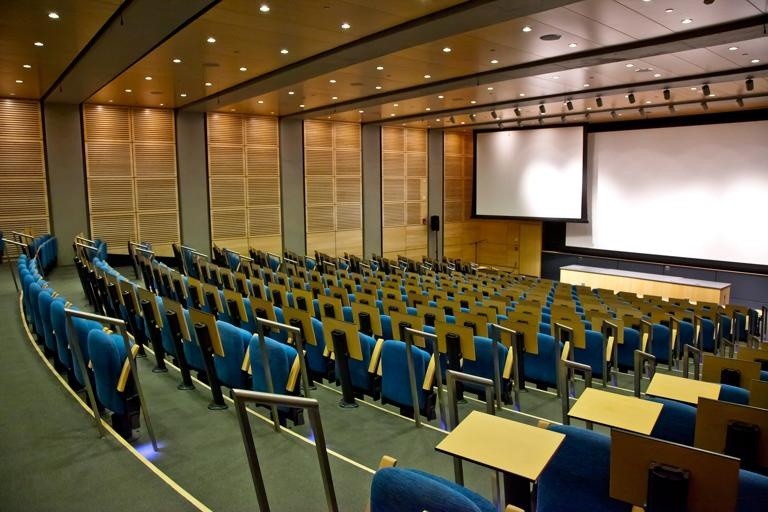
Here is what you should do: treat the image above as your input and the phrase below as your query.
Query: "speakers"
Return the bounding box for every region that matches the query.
[431,215,440,232]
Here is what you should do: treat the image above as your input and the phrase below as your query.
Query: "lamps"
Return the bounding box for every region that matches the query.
[514,107,520,116]
[539,119,545,126]
[491,108,497,119]
[746,78,753,91]
[585,113,591,119]
[518,120,522,127]
[450,117,455,123]
[540,103,545,113]
[736,98,744,107]
[702,84,710,96]
[566,100,574,110]
[595,96,602,107]
[669,105,675,113]
[628,92,635,104]
[611,111,617,118]
[562,116,567,121]
[639,108,645,116]
[469,114,475,122]
[498,123,504,128]
[702,102,708,112]
[663,88,669,99]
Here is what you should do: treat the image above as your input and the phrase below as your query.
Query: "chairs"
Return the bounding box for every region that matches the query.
[17,234,768,512]
